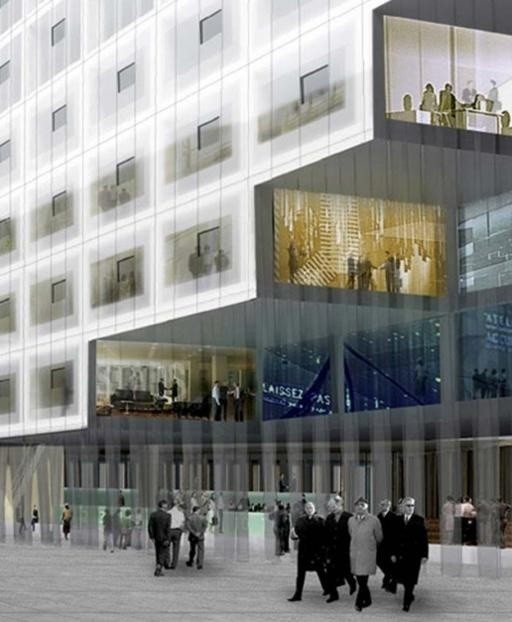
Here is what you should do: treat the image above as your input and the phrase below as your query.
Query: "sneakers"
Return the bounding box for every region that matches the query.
[155,560,203,576]
[287,594,302,600]
[321,577,414,611]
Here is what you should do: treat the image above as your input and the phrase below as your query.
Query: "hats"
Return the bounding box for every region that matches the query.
[353,496,369,506]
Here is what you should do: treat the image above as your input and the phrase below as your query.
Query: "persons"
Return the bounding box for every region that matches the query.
[14,489,225,577]
[96,263,141,303]
[472,365,508,400]
[346,240,443,297]
[290,493,428,614]
[443,495,510,546]
[288,241,307,281]
[183,240,228,278]
[157,376,248,422]
[97,180,128,207]
[413,358,429,395]
[417,80,501,121]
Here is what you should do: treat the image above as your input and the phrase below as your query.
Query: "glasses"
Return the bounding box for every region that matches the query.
[405,504,414,507]
